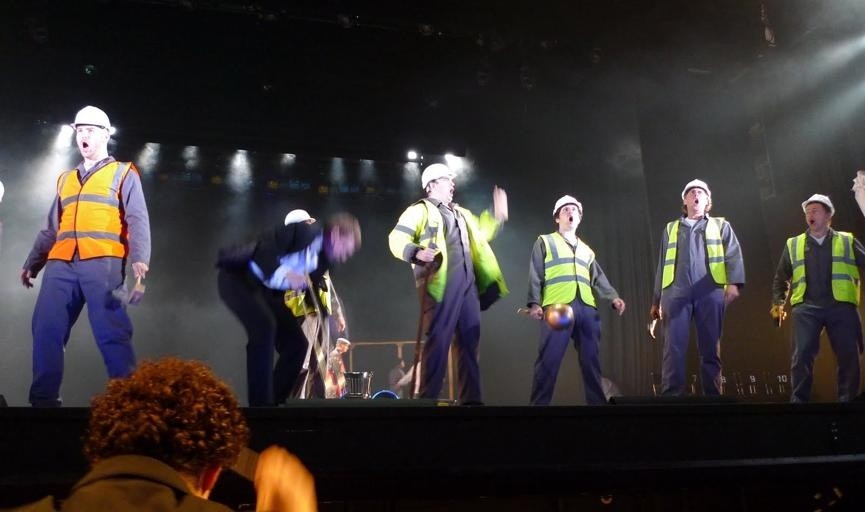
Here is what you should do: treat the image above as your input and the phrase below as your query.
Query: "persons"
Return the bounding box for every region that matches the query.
[649,181,744,398]
[394,351,423,400]
[284,209,348,357]
[526,195,626,405]
[389,164,508,403]
[391,360,406,397]
[772,193,865,403]
[15,358,316,511]
[18,106,152,409]
[327,339,351,397]
[213,212,360,406]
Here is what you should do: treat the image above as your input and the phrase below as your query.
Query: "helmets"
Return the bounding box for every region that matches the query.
[73,107,112,132]
[420,163,461,185]
[283,208,317,224]
[801,193,838,218]
[681,179,713,199]
[554,194,585,216]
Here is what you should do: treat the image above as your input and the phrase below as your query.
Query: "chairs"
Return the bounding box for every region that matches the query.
[342,366,370,399]
